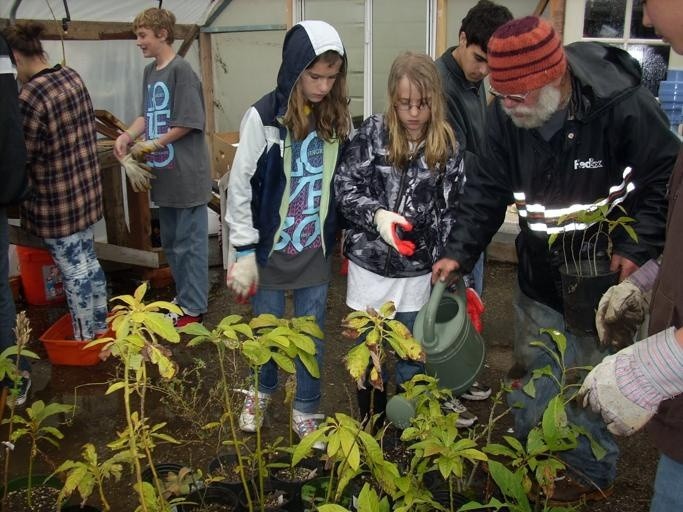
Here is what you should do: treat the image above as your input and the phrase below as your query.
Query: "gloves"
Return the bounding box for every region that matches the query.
[130,140,163,162]
[226,253,259,302]
[595,257,660,350]
[453,287,485,331]
[120,153,158,194]
[374,209,416,257]
[576,326,682,438]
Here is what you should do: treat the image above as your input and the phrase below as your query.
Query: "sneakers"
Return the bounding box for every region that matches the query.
[461,381,492,401]
[291,408,328,451]
[526,473,614,506]
[6,375,32,406]
[163,297,205,334]
[232,384,271,432]
[440,397,479,431]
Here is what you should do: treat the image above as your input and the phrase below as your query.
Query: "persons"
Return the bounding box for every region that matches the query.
[329,46,469,454]
[431,1,515,428]
[432,17,683,511]
[1,74,33,408]
[222,19,355,458]
[573,1,683,512]
[0,21,107,341]
[113,6,214,336]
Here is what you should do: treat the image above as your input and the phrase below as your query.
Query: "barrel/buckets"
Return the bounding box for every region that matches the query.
[15,245,69,305]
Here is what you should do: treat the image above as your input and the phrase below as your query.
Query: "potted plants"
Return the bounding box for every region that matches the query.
[0,197,640,512]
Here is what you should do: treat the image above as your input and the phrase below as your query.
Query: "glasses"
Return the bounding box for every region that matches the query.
[397,97,432,111]
[488,87,529,103]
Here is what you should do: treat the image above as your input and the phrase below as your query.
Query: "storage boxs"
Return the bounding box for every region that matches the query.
[213,132,241,178]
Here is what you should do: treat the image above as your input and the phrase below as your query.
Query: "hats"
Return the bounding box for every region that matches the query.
[486,16,567,94]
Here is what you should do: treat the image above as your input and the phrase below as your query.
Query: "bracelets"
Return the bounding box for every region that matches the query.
[126,130,136,140]
[122,132,132,144]
